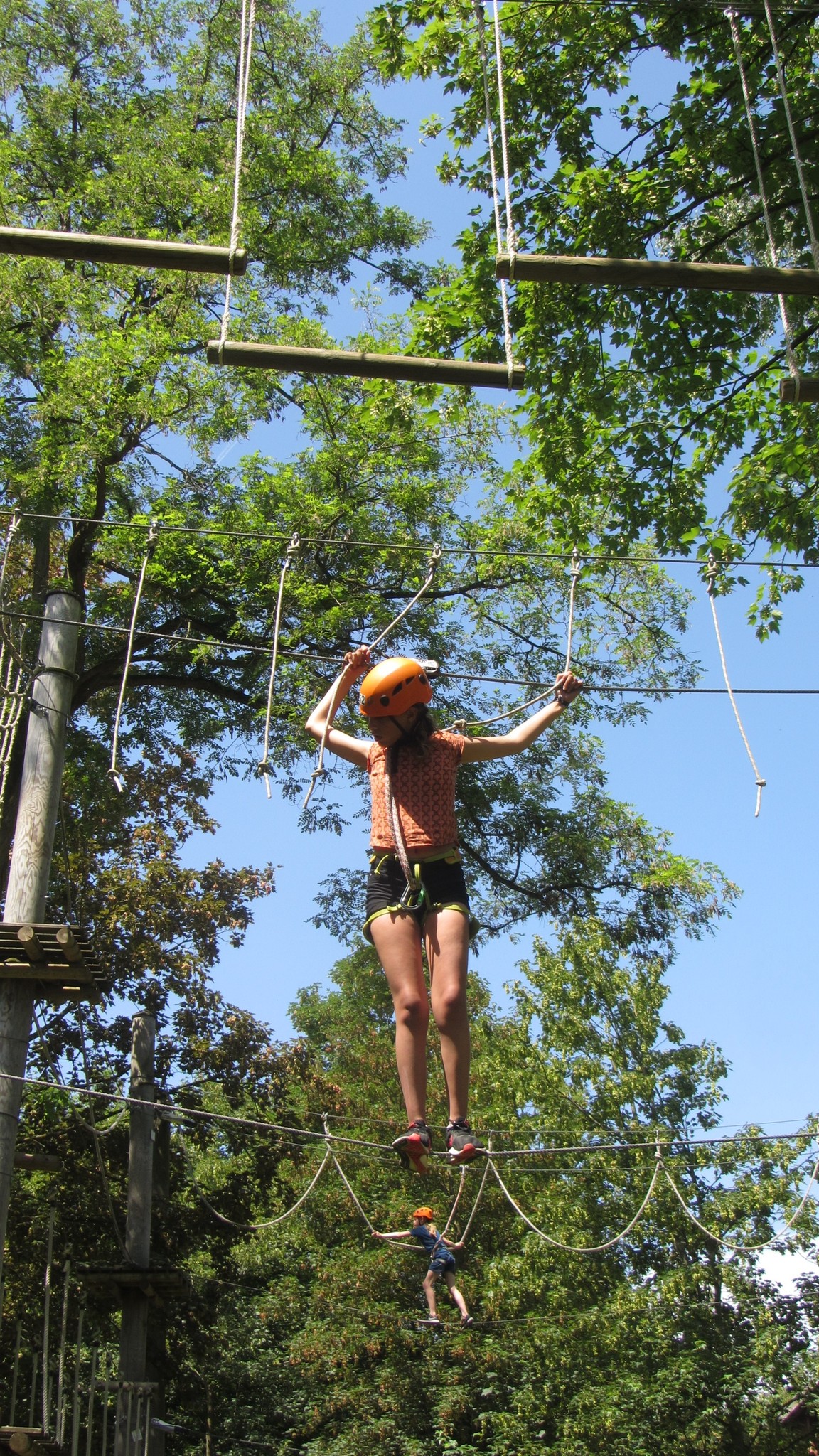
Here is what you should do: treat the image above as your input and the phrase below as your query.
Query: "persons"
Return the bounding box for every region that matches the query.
[371,1207,474,1331]
[305,646,585,1173]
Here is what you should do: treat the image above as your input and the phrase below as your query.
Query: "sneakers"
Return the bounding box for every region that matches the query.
[417,1315,440,1323]
[392,1119,432,1173]
[460,1315,474,1330]
[446,1117,484,1164]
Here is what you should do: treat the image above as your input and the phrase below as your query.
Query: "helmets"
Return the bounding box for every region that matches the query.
[413,1207,433,1219]
[359,657,432,717]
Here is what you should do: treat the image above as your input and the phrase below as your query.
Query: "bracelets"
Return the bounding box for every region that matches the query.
[553,695,570,707]
